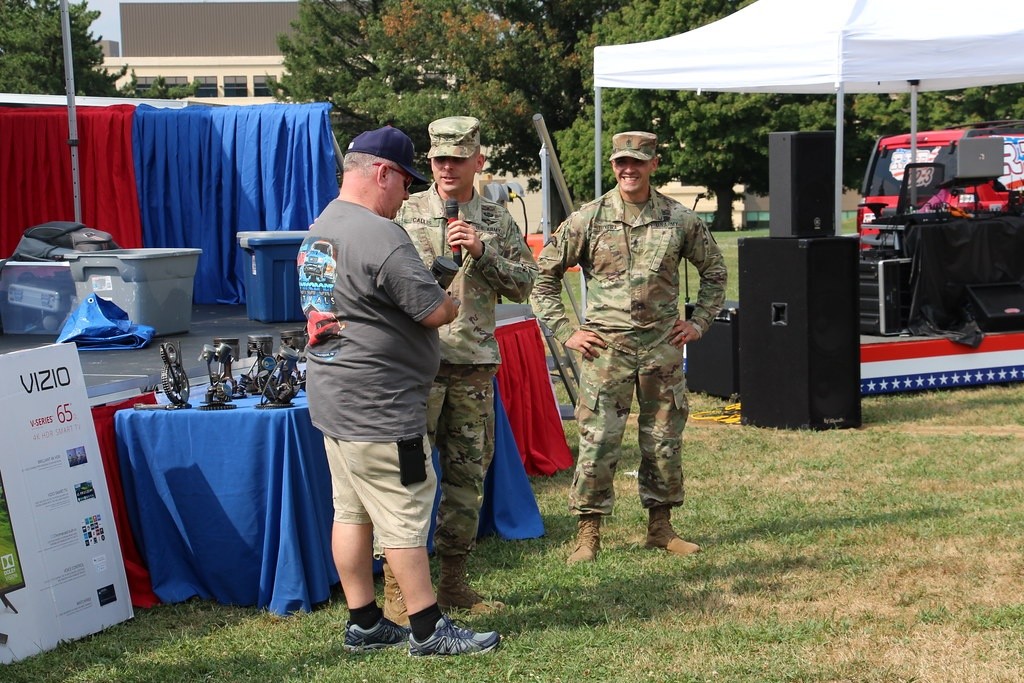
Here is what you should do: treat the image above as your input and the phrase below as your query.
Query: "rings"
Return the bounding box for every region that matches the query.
[464,234,467,239]
[682,337,687,343]
[681,330,687,335]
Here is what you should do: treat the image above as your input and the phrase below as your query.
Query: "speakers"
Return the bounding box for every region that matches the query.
[687,131,862,432]
[933,136,1005,188]
[965,280,1024,333]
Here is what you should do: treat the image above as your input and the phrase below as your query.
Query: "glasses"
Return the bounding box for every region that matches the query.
[373,162,414,192]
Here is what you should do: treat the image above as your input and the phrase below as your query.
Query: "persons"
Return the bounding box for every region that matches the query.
[529,131,728,564]
[309,116,539,628]
[298,126,501,657]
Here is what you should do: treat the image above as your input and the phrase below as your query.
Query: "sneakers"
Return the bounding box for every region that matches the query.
[408,612,500,659]
[343,608,412,652]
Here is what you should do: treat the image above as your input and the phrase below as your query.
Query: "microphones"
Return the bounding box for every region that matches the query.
[444,198,462,266]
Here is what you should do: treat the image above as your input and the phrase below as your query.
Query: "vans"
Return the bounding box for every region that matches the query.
[856,119,1024,253]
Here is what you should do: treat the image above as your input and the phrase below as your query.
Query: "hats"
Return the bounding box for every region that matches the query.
[343,125,430,186]
[609,131,658,161]
[427,116,480,159]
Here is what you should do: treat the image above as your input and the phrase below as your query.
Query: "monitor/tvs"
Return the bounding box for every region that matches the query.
[0,470,26,596]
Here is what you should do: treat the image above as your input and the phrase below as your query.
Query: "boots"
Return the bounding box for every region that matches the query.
[382,556,414,634]
[645,506,701,555]
[437,553,505,615]
[566,513,602,565]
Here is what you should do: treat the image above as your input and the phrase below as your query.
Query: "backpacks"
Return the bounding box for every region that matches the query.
[0,221,121,282]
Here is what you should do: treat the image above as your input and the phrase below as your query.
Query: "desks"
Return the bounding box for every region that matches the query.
[114,316,575,616]
[872,211,1024,348]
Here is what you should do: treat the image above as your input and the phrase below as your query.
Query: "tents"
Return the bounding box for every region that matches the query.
[595,1,1023,236]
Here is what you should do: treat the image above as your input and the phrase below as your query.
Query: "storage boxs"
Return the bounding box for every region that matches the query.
[63,248,203,338]
[238,229,313,323]
[0,257,73,335]
